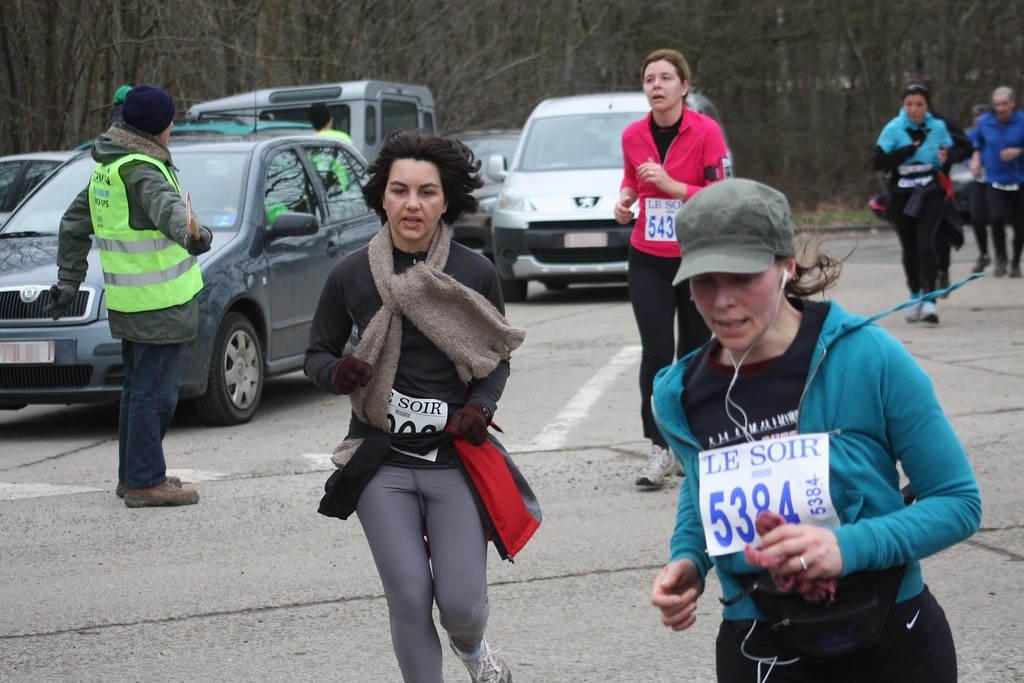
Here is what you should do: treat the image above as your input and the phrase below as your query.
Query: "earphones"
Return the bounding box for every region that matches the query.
[781,269,787,290]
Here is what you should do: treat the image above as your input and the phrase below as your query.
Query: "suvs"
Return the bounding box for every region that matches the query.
[440,128,526,246]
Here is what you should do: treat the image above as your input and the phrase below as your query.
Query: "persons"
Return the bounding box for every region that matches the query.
[224,198,289,228]
[873,87,973,323]
[43,86,213,507]
[650,178,982,683]
[614,51,729,485]
[964,87,1024,277]
[302,133,526,683]
[308,102,353,192]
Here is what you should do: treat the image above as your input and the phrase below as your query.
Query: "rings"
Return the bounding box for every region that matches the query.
[649,171,654,176]
[800,556,807,570]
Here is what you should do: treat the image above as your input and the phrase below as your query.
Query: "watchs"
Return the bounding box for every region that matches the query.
[482,405,491,417]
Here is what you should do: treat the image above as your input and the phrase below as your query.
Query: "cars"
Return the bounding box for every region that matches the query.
[0,150,86,232]
[0,128,385,427]
[79,118,313,138]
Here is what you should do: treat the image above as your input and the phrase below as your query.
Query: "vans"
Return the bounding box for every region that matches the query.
[486,93,732,300]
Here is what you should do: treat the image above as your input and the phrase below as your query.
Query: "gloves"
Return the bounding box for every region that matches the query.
[42,280,77,322]
[184,226,212,258]
[450,402,488,447]
[330,354,374,395]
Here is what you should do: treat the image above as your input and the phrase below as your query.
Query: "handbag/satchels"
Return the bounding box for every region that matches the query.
[718,561,909,669]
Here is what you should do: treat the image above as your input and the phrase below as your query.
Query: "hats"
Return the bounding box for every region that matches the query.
[114,84,132,104]
[123,86,176,136]
[672,179,795,286]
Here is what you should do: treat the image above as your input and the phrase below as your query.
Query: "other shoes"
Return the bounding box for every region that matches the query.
[994,258,1008,278]
[919,301,938,324]
[936,274,948,298]
[1011,264,1020,279]
[971,255,991,274]
[904,294,921,322]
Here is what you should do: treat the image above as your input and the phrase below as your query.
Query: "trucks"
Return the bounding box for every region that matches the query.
[186,79,439,182]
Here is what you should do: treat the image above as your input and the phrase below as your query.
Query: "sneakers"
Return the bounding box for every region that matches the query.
[448,632,511,683]
[123,483,200,507]
[672,453,686,476]
[116,476,183,497]
[634,444,671,488]
